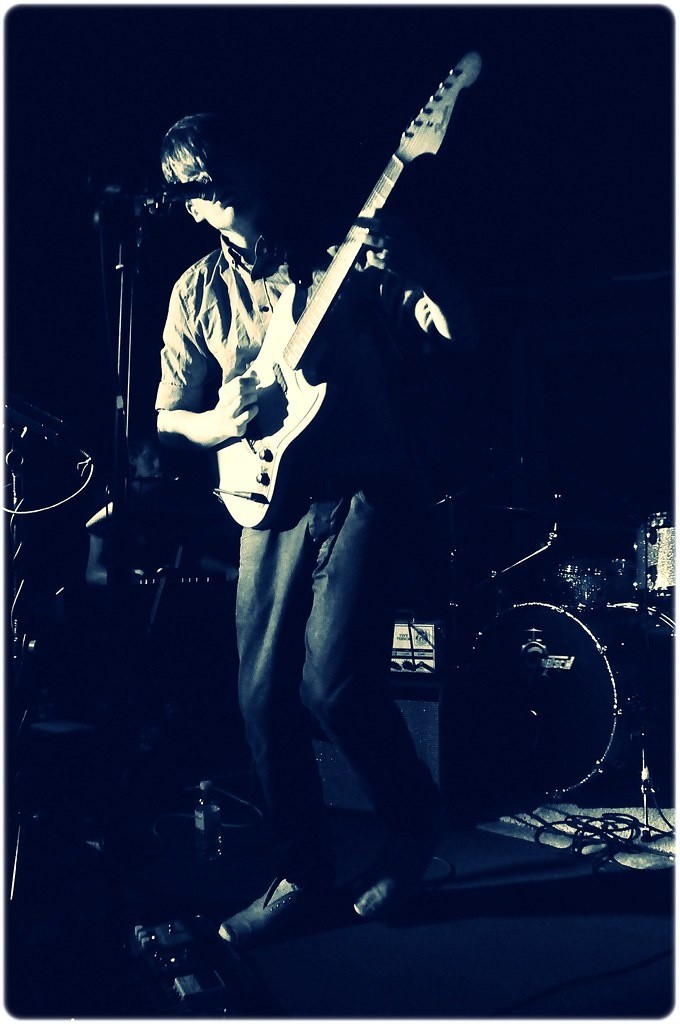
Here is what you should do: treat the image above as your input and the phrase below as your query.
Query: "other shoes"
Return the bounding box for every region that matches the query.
[218,873,331,947]
[353,876,421,917]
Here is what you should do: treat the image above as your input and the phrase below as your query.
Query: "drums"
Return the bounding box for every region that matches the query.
[557,549,625,610]
[632,507,675,601]
[468,600,674,808]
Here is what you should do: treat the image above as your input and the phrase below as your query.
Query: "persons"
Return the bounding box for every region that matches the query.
[153,111,494,945]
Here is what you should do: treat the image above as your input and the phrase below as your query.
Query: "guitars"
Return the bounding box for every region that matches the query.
[216,50,486,535]
